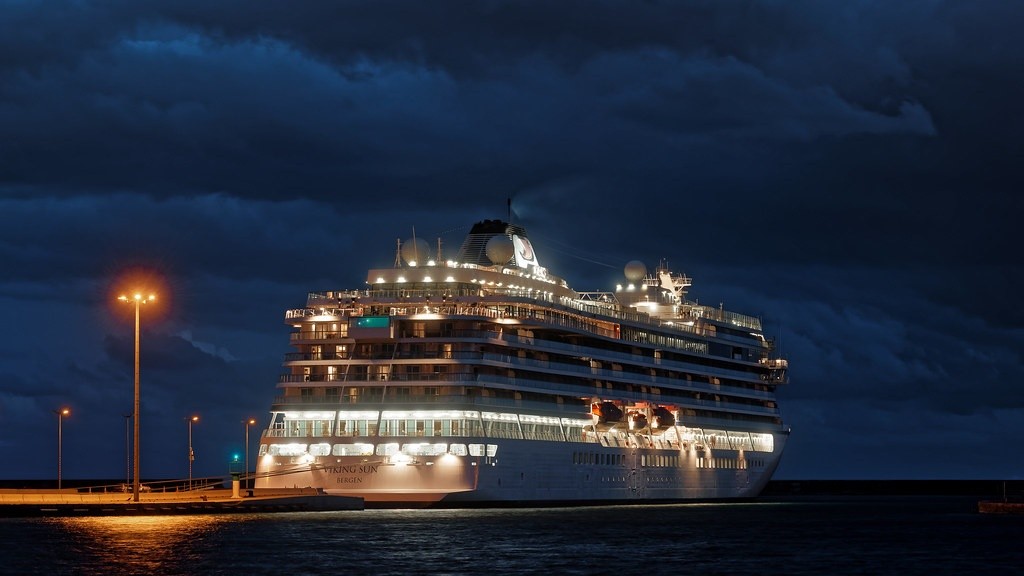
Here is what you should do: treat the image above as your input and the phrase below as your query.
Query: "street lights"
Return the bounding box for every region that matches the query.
[116,293,157,501]
[245,420,255,490]
[58,409,68,490]
[188,416,198,491]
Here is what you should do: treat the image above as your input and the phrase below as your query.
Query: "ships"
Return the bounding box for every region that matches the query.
[250,196,794,505]
